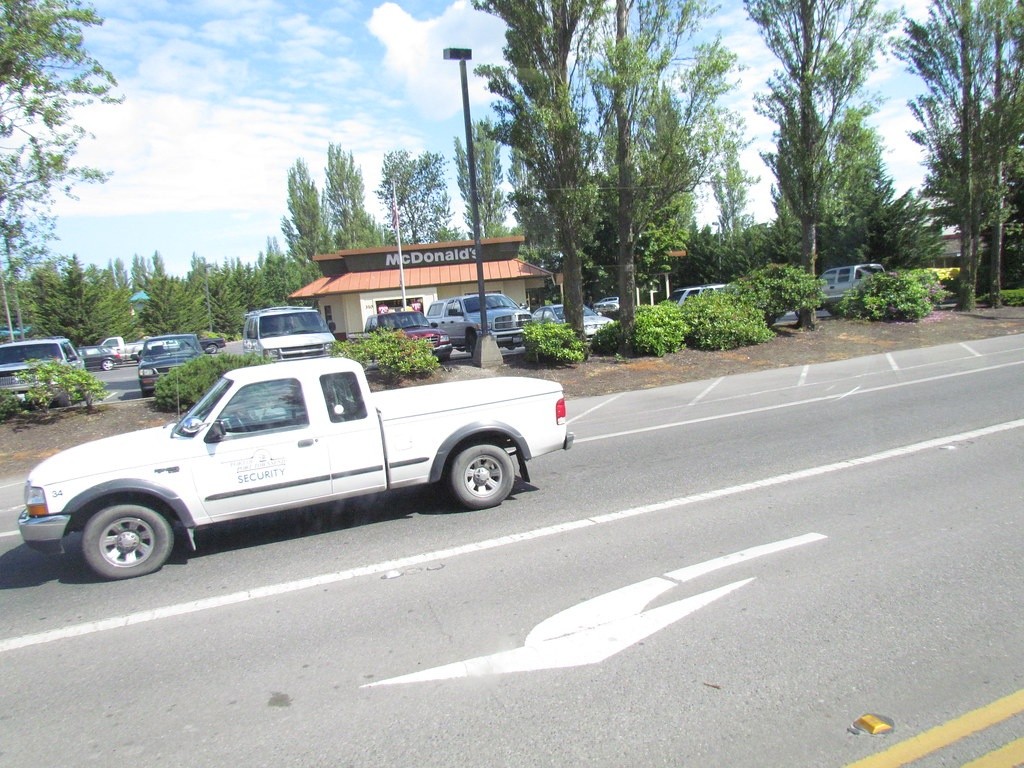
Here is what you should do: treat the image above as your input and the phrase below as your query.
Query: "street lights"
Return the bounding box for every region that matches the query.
[443,48,503,367]
[205,264,213,333]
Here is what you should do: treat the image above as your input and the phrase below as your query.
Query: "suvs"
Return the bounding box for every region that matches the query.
[426,294,543,358]
[0,336,87,410]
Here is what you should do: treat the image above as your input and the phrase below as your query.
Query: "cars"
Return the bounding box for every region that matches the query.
[129,334,214,397]
[531,303,614,346]
[592,296,620,314]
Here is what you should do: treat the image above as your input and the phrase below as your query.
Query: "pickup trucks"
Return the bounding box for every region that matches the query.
[348,311,453,361]
[74,336,226,371]
[18,357,574,581]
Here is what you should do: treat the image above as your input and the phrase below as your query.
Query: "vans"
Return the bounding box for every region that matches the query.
[667,284,736,308]
[243,306,338,363]
[815,264,886,310]
